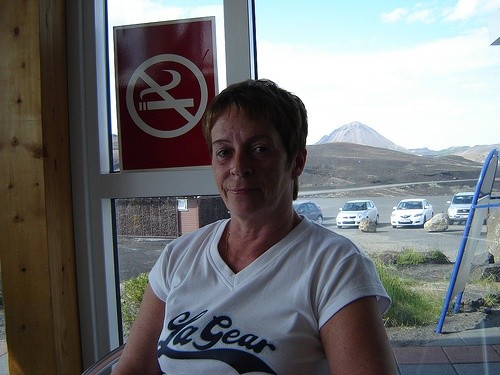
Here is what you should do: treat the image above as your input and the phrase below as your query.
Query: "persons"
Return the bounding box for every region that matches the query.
[114,77,399,374]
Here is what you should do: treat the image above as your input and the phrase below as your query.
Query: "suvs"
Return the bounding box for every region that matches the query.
[447,192,474,225]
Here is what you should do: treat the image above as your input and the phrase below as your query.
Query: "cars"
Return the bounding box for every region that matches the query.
[293,202,323,224]
[390,199,434,228]
[335,200,379,229]
